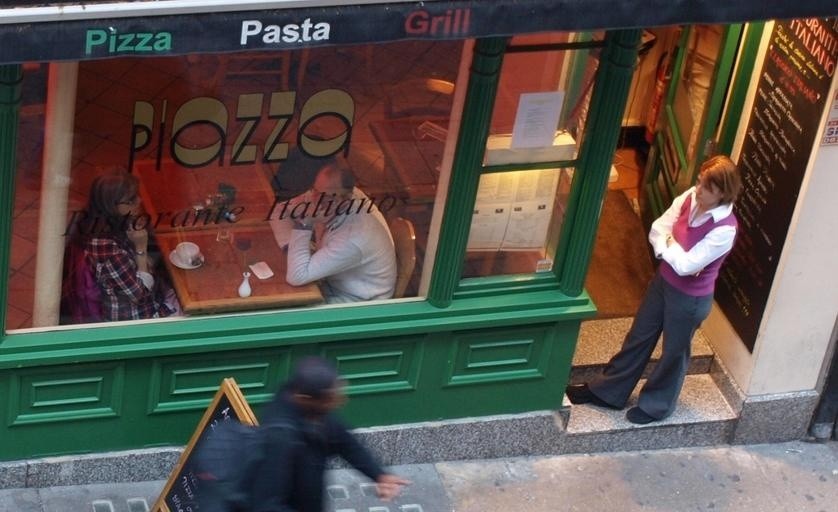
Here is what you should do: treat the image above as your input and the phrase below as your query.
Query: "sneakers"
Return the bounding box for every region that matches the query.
[625,405,656,425]
[566,383,593,404]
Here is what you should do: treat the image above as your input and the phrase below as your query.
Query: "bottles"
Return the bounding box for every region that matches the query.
[239,272,254,298]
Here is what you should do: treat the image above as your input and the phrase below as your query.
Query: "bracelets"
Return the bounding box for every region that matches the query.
[294,221,312,229]
[135,250,146,255]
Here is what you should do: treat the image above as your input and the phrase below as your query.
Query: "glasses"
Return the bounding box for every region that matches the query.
[118,192,138,206]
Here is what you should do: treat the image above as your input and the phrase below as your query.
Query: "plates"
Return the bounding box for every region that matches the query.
[170,251,205,269]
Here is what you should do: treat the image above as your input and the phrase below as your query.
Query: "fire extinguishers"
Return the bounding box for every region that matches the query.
[643,46,680,145]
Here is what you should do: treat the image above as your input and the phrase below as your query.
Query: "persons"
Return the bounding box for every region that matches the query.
[192,420,271,511]
[75,171,181,322]
[261,360,411,512]
[269,163,397,306]
[565,155,742,424]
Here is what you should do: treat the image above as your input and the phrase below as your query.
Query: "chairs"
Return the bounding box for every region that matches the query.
[388,216,414,298]
[211,51,292,97]
[270,144,343,202]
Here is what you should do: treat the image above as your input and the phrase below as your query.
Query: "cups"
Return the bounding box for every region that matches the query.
[176,241,199,264]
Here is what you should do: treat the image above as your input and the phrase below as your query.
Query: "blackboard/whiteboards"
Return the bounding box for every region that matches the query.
[713,15,838,354]
[160,376,260,512]
